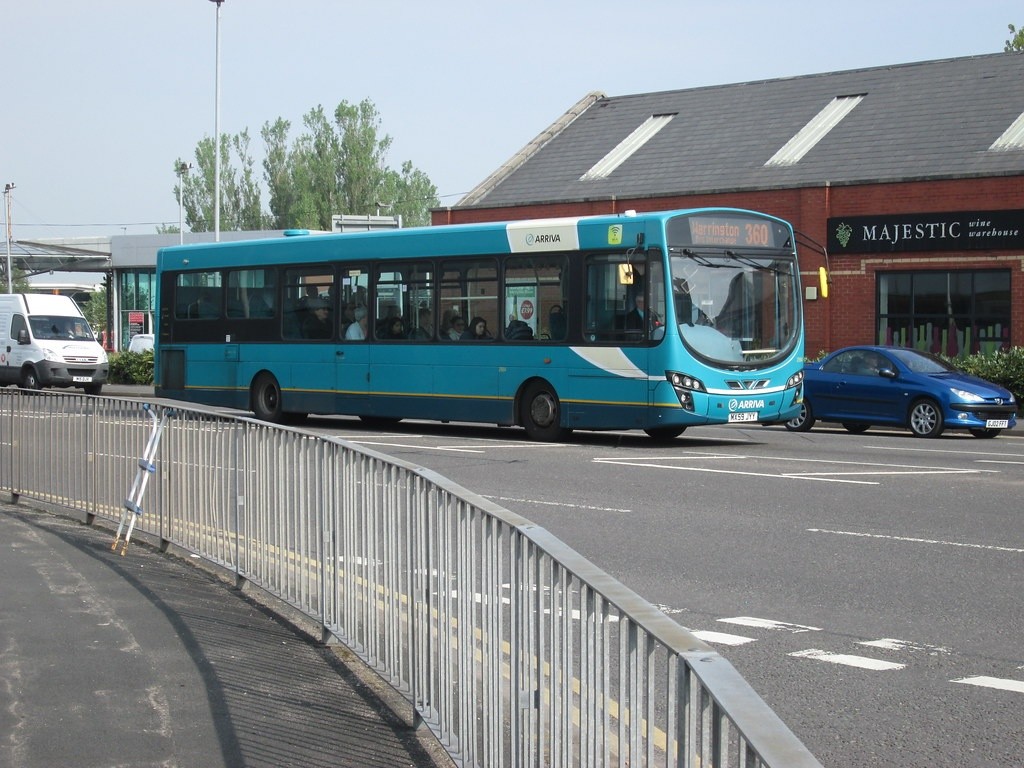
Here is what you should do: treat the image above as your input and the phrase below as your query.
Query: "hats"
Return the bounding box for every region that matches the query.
[305,297,332,310]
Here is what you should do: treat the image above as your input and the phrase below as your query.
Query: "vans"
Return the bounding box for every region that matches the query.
[128,333,155,355]
[0,292,109,396]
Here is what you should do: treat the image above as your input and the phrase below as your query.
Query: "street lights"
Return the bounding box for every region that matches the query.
[1,182,18,293]
[208,0,227,286]
[178,163,194,286]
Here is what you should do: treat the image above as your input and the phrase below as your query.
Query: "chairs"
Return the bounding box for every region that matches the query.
[409,324,491,339]
[849,356,867,372]
[550,303,568,339]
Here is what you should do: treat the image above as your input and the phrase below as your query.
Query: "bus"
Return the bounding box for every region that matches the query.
[154,199,832,443]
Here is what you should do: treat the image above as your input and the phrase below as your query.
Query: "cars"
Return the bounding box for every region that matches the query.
[782,343,1019,440]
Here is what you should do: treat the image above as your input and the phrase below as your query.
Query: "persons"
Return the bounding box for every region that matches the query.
[296,283,527,345]
[857,352,880,376]
[625,289,661,339]
